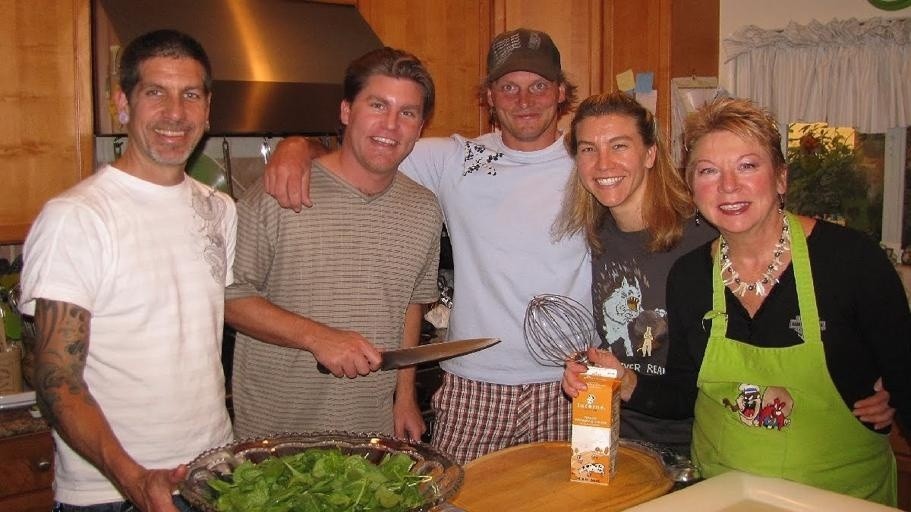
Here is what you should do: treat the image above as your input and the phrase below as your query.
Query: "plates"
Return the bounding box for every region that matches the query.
[177,425,464,512]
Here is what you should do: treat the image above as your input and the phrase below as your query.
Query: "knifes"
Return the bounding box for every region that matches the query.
[316,340,501,375]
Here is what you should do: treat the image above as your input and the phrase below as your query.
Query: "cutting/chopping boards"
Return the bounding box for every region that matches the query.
[428,438,675,512]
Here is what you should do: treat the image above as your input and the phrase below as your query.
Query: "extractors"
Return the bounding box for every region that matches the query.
[88,1,387,136]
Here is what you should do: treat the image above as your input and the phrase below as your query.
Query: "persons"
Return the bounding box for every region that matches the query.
[549,90,897,469]
[263,30,603,468]
[17,30,238,512]
[562,96,911,509]
[223,47,443,443]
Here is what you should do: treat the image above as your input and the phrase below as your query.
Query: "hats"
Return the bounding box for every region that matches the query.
[484,29,562,84]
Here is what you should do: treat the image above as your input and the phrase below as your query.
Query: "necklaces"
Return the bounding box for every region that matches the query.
[718,208,791,298]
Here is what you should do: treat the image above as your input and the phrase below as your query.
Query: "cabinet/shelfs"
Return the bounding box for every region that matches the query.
[351,1,723,173]
[0,430,58,511]
[0,1,96,249]
